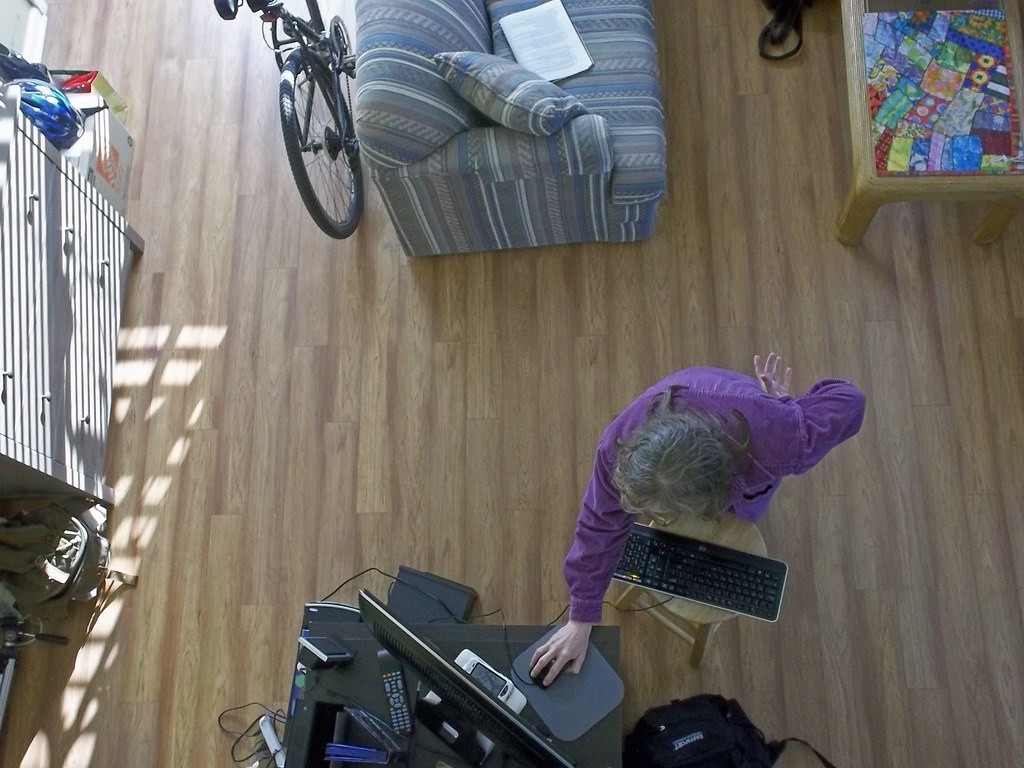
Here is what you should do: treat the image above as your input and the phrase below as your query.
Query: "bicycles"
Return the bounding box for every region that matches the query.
[214,0,366,241]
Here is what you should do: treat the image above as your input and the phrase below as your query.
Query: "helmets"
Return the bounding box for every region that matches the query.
[0,79,85,149]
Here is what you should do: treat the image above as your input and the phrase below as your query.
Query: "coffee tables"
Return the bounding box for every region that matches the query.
[835,0,1024,247]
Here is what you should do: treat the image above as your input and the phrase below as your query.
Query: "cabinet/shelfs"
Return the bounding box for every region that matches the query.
[0,82,145,515]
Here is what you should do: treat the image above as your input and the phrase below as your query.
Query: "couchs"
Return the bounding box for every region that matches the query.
[353,0,668,257]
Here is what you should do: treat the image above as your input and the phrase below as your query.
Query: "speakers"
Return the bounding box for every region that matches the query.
[298,636,353,670]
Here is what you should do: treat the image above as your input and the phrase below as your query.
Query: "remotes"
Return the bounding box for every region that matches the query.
[377,648,414,737]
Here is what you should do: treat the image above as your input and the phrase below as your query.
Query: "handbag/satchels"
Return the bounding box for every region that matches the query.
[758,0,813,60]
[621,694,772,768]
[0,43,50,83]
[48,70,128,125]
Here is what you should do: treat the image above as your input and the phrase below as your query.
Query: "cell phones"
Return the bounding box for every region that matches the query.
[462,657,514,702]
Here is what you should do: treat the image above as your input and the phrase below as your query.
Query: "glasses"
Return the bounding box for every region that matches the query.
[644,510,679,527]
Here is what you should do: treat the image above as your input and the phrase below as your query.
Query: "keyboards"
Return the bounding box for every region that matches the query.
[611,523,788,621]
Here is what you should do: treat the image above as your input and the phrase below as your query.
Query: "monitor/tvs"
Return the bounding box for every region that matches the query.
[358,589,577,768]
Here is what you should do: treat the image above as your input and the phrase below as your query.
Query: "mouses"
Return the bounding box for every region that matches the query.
[530,657,573,690]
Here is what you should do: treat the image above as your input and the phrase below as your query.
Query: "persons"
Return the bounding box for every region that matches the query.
[528,352,866,686]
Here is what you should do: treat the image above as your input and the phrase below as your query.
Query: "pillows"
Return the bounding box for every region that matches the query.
[434,53,588,137]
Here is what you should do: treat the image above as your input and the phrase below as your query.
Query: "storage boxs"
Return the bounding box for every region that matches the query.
[57,82,134,218]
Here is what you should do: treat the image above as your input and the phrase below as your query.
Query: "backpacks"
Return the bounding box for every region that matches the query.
[0,488,138,617]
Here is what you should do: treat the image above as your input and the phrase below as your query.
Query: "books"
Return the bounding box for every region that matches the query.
[499,0,595,84]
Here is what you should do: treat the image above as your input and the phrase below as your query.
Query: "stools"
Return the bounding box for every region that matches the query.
[614,515,766,669]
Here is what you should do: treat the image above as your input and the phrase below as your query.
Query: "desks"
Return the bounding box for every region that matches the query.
[285,620,623,768]
